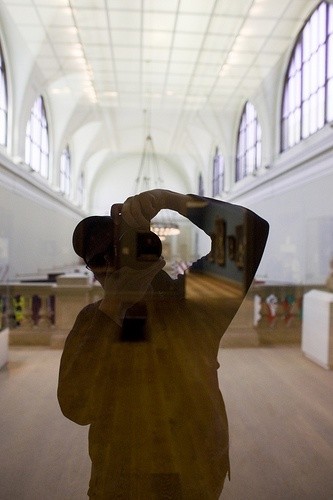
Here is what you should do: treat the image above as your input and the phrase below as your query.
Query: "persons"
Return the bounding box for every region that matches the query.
[56,188,270,500]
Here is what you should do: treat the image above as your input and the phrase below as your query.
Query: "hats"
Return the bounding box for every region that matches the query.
[71,215,114,259]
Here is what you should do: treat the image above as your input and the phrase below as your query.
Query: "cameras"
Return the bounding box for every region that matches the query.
[114,209,162,268]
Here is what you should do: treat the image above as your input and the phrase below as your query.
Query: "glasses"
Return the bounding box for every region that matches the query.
[86,241,114,270]
